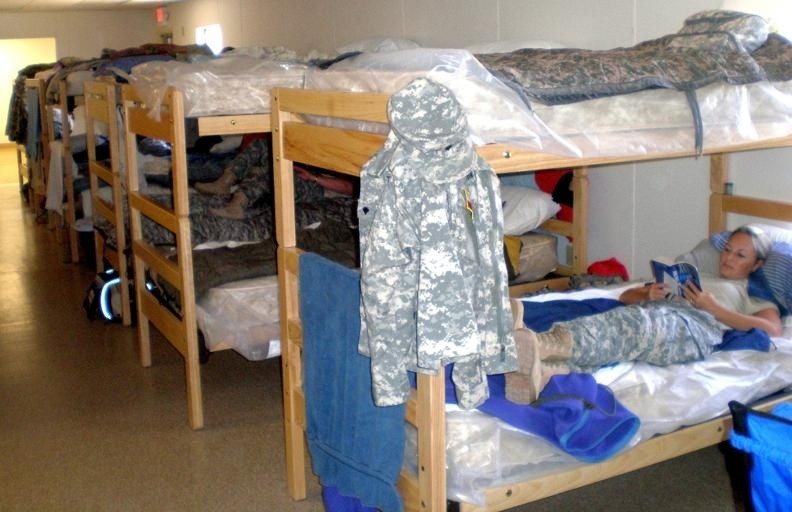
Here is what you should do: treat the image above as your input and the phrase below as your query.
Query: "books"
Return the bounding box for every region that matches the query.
[650,255,702,298]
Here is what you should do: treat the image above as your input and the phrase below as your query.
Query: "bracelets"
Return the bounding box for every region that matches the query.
[313,175,319,183]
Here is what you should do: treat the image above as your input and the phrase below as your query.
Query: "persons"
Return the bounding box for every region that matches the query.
[504,223,783,406]
[193,136,355,220]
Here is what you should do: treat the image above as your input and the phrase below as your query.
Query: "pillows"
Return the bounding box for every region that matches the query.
[677,10,771,53]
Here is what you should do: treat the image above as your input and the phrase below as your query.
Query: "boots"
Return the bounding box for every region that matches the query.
[195,168,237,195]
[504,297,573,405]
[208,191,250,220]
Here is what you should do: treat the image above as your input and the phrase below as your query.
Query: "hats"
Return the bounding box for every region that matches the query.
[386,77,478,185]
